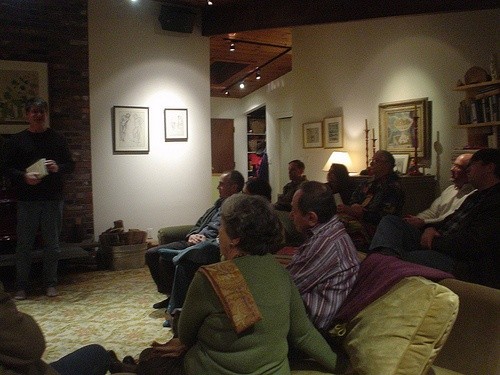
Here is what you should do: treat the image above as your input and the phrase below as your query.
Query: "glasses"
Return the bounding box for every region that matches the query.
[369,159,384,163]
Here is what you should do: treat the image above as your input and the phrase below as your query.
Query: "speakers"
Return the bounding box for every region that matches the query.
[158,4,197,33]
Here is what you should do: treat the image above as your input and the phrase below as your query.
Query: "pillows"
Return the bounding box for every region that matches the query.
[329,263,459,375]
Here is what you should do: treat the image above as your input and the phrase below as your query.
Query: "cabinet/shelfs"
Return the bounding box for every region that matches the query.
[247,118,265,181]
[452,82,500,150]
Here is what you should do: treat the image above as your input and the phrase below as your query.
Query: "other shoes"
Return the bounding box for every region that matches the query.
[13,287,26,299]
[163,321,170,326]
[153,296,170,309]
[46,287,58,296]
[123,356,134,364]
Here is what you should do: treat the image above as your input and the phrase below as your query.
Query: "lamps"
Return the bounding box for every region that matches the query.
[255,67,261,80]
[229,42,236,51]
[321,152,357,172]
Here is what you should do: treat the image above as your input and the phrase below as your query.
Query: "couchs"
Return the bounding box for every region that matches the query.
[289,254,500,375]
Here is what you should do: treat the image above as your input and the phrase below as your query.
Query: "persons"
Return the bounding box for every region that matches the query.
[133,193,356,375]
[336,149,404,251]
[273,159,309,211]
[409,158,416,173]
[324,164,352,205]
[281,181,360,329]
[0,281,111,375]
[0,97,74,300]
[367,147,499,290]
[163,178,272,326]
[143,171,244,306]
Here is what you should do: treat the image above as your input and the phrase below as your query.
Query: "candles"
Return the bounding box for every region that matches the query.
[372,128,375,138]
[365,118,368,129]
[415,102,417,116]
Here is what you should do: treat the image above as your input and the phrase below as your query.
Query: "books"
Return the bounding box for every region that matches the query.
[458,87,500,148]
[26,158,48,179]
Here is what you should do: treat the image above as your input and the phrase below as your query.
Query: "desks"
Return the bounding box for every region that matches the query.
[346,175,435,225]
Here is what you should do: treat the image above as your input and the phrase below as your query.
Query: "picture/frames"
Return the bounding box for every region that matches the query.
[377,97,430,155]
[0,58,52,136]
[164,108,189,141]
[303,117,344,148]
[113,106,150,154]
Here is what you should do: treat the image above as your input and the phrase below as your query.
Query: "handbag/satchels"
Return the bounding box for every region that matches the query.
[137,337,189,375]
